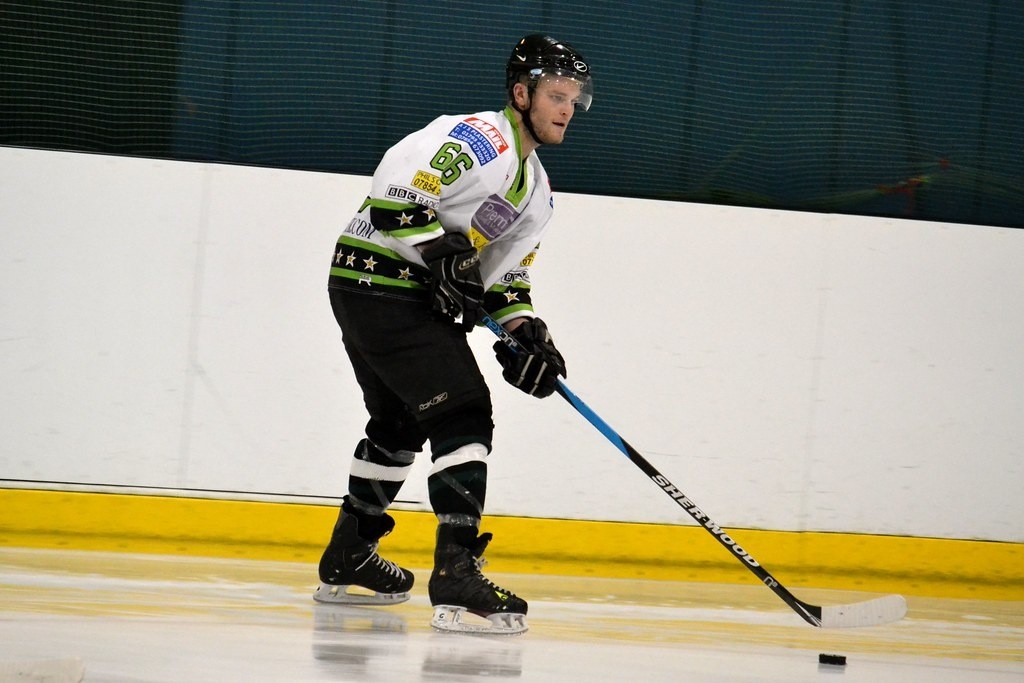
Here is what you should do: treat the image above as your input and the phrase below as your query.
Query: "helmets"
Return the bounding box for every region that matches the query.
[506,34,590,91]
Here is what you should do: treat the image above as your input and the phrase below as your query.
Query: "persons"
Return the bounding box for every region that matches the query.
[313,34,595,639]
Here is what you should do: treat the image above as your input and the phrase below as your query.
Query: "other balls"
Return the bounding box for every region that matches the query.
[819,653,847,666]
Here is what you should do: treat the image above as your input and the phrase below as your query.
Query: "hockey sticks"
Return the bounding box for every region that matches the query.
[479,306,908,628]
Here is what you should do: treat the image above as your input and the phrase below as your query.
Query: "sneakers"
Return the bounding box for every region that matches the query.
[314,495,416,606]
[428,524,529,633]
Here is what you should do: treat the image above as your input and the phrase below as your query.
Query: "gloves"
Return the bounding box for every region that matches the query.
[421,232,484,331]
[492,317,568,399]
